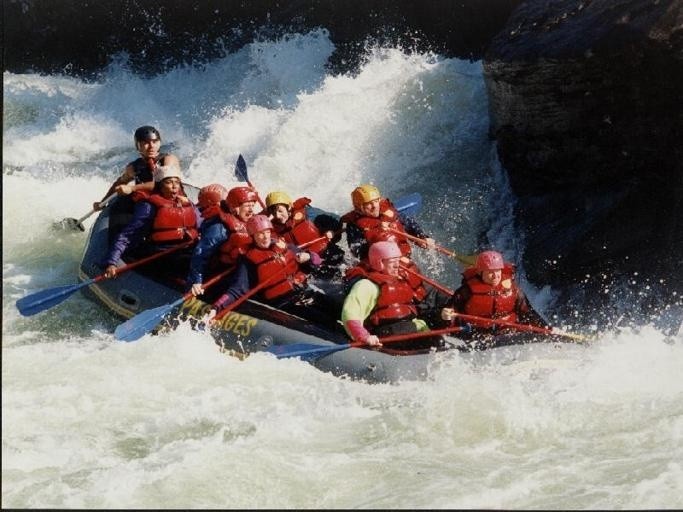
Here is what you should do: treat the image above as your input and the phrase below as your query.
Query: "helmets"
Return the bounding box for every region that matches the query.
[155,165,180,183]
[477,251,504,270]
[352,185,380,211]
[199,184,291,235]
[369,241,402,271]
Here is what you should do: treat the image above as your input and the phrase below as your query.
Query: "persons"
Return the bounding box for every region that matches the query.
[432,252,560,342]
[338,183,435,274]
[91,125,348,337]
[342,241,432,351]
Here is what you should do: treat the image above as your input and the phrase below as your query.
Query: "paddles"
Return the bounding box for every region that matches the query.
[447,307,593,346]
[54,191,118,232]
[16,237,201,316]
[389,226,478,266]
[234,155,267,212]
[114,264,240,342]
[298,192,422,250]
[266,322,471,361]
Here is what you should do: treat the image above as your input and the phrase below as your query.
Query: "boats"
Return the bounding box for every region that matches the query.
[75,181,586,383]
